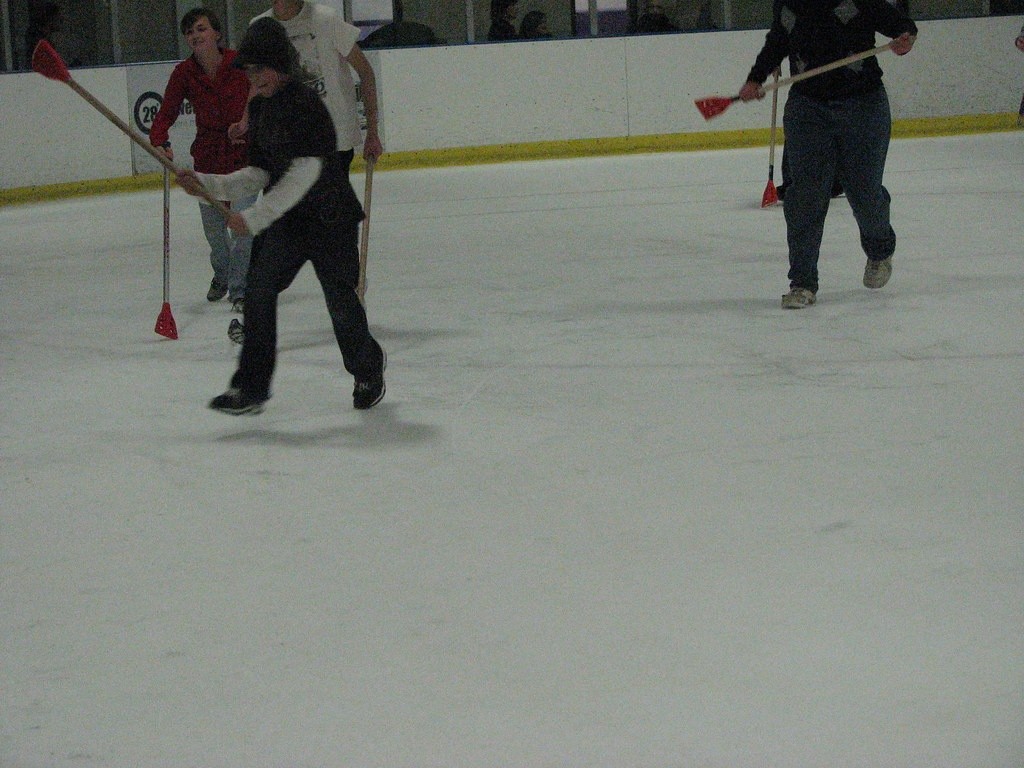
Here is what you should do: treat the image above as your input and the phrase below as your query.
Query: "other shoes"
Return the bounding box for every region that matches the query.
[774,184,785,205]
[227,311,249,344]
[207,275,228,301]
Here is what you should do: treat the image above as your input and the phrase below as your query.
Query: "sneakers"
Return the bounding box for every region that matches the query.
[782,287,814,307]
[862,253,892,288]
[208,388,272,415]
[353,342,388,410]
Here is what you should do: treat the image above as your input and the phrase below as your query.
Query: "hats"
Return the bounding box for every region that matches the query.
[232,17,288,68]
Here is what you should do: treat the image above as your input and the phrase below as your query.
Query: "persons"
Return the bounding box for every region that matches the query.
[740,0,918,310]
[149,8,258,310]
[174,17,385,413]
[490,0,555,43]
[228,0,384,222]
[632,0,678,31]
